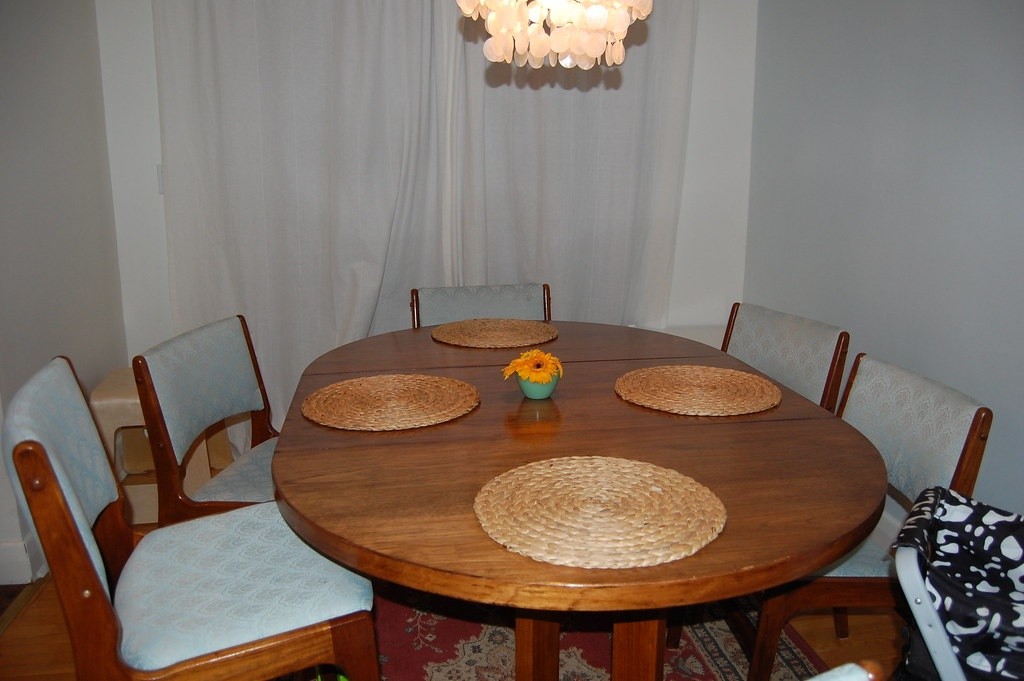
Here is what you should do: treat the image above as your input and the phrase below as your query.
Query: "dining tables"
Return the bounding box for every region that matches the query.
[271,317,889,681]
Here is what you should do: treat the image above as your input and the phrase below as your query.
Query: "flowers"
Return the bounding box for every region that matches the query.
[500,347,563,384]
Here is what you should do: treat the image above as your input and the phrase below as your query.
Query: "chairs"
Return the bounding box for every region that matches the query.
[721,301,850,414]
[0,355,380,681]
[666,353,993,681]
[409,282,551,328]
[132,314,281,528]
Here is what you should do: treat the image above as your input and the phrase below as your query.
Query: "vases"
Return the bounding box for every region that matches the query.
[517,372,559,399]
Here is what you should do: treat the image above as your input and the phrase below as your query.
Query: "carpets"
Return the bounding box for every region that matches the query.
[373,586,831,681]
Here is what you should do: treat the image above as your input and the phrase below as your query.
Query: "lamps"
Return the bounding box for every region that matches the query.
[456,0,653,70]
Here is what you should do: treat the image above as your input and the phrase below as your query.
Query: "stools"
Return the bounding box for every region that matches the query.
[89,366,235,524]
